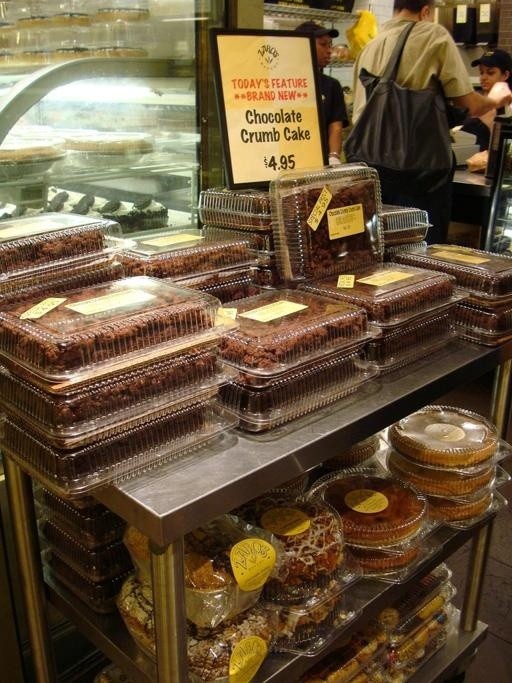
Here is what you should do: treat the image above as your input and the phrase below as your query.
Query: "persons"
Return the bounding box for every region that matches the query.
[352,0,512,247]
[293,21,351,167]
[461,49,512,153]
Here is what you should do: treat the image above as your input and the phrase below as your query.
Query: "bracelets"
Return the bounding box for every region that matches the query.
[327,152,341,159]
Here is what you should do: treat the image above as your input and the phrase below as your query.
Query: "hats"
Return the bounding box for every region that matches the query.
[472,50,511,70]
[293,21,337,40]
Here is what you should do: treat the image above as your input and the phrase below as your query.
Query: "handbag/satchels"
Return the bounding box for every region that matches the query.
[344,68,453,181]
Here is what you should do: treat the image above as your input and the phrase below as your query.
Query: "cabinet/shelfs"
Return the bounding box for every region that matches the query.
[0,3,511,680]
